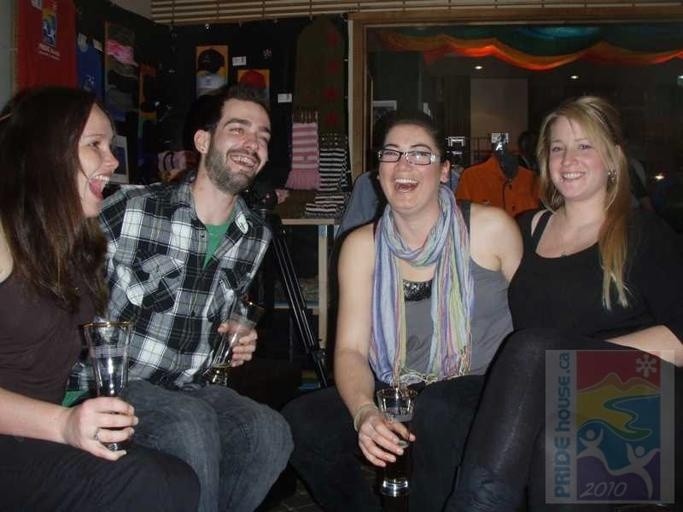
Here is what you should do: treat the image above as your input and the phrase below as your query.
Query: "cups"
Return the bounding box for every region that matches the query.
[86,322,132,452]
[376,388,417,497]
[202,297,265,387]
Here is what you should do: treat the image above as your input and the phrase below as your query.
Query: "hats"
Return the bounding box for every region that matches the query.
[239,70,265,90]
[197,48,225,74]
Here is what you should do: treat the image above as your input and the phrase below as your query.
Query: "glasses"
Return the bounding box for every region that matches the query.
[378,149,443,164]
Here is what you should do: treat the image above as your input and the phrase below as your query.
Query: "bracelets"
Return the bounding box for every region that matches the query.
[353,404,378,432]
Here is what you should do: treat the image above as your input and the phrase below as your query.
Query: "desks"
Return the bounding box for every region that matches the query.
[269,217,345,370]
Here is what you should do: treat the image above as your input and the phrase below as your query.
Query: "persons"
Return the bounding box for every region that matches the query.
[445,97,683,512]
[627,161,655,209]
[279,108,524,511]
[0,86,200,512]
[517,130,539,171]
[63,83,294,512]
